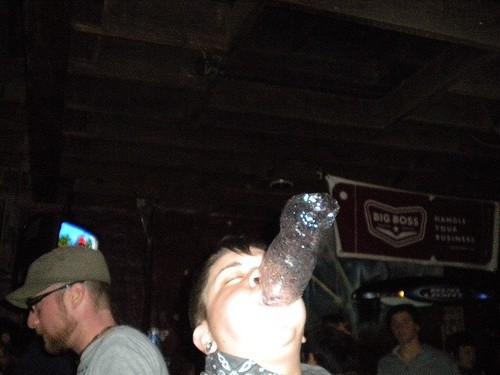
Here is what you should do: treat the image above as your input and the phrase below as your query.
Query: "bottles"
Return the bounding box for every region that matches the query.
[259,192,340,307]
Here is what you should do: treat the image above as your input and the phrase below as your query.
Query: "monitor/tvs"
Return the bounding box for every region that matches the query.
[15,214,100,267]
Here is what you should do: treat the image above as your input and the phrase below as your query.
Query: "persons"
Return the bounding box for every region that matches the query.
[303,304,500,375]
[188,233,331,375]
[4,246,169,375]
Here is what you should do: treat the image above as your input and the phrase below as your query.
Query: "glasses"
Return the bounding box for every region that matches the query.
[389,318,414,329]
[25,279,83,312]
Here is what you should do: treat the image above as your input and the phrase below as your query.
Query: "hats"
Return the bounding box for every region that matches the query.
[6,246,109,308]
